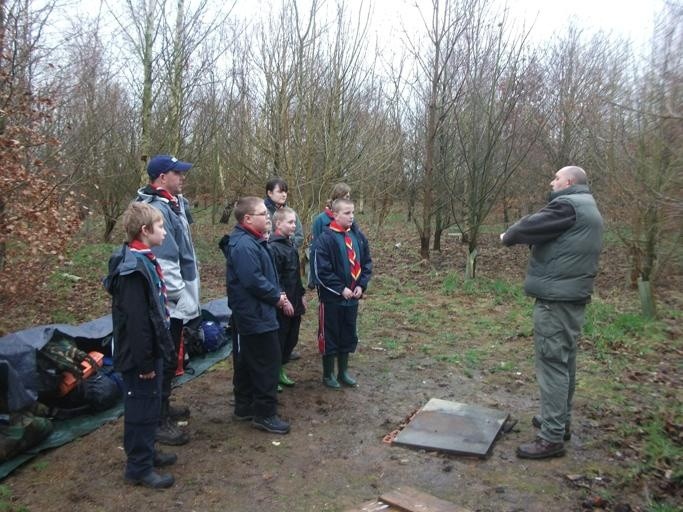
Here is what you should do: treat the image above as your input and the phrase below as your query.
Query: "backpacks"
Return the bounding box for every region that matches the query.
[0,330,124,464]
[183,320,229,356]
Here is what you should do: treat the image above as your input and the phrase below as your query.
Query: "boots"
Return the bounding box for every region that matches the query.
[336,353,357,386]
[322,354,341,389]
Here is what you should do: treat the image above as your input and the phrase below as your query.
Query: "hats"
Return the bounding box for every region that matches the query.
[146,155,194,180]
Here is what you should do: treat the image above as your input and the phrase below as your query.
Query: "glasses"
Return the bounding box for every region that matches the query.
[248,210,267,217]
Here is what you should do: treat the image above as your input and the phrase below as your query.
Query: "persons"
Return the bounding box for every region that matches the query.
[500,165,602,460]
[218,196,291,435]
[306,182,351,354]
[264,211,271,243]
[264,178,305,248]
[135,154,202,447]
[310,198,372,388]
[105,203,178,489]
[267,206,306,392]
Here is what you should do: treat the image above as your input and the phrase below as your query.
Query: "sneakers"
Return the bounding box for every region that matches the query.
[155,417,190,446]
[153,448,177,467]
[531,414,571,441]
[125,469,173,488]
[515,435,565,459]
[168,404,190,420]
[233,351,300,434]
